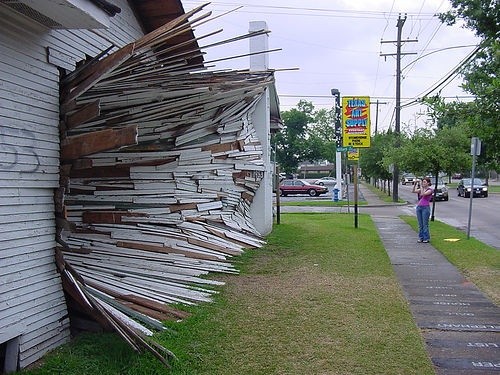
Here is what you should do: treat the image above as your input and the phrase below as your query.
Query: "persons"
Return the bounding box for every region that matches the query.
[412,178,433,243]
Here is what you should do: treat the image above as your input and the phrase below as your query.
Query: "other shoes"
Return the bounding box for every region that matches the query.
[417,239,423,242]
[423,240,429,243]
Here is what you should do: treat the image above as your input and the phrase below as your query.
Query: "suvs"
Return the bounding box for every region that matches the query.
[402,173,416,185]
[417,176,449,201]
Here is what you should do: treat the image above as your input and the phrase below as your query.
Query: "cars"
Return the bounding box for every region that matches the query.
[315,177,345,186]
[272,180,329,197]
[457,178,488,198]
[452,173,463,179]
